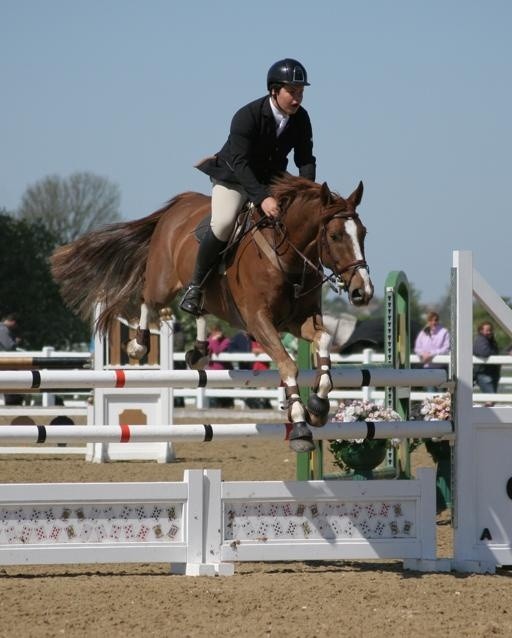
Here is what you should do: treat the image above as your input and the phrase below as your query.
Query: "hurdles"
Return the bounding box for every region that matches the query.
[0,369,451,578]
[450,251,511,580]
[0,284,175,465]
[294,268,451,514]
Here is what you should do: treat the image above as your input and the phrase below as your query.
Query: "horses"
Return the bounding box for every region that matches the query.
[48,170,374,452]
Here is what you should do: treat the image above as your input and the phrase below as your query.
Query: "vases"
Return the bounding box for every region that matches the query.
[423,438,457,508]
[329,438,386,481]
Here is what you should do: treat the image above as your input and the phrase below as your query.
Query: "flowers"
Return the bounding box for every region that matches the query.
[409,392,496,443]
[328,399,405,450]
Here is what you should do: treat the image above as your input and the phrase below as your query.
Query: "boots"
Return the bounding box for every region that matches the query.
[181,224,228,313]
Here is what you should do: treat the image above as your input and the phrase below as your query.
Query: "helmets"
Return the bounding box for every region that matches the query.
[267,57,310,87]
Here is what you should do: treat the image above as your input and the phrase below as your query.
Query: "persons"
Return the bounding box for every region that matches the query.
[206,322,231,370]
[0,312,20,352]
[411,312,453,390]
[226,329,257,373]
[468,322,504,398]
[249,337,275,410]
[179,59,317,318]
